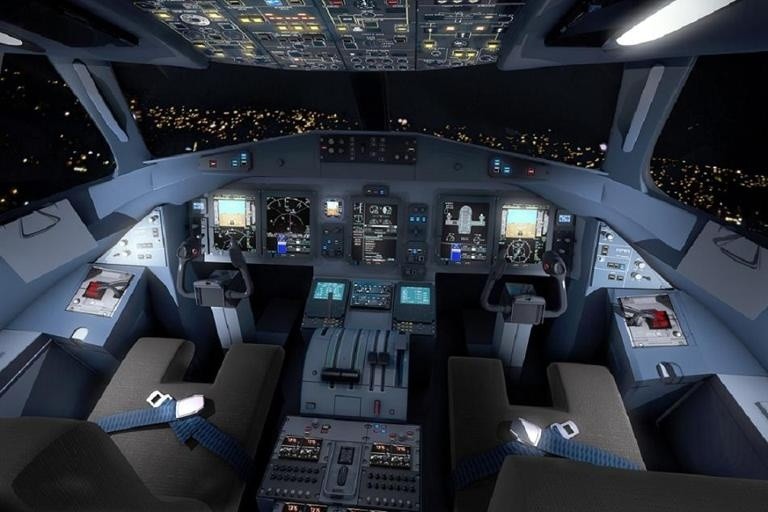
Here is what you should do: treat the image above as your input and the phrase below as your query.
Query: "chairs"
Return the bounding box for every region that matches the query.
[0,339,293,512]
[444,351,767,511]
